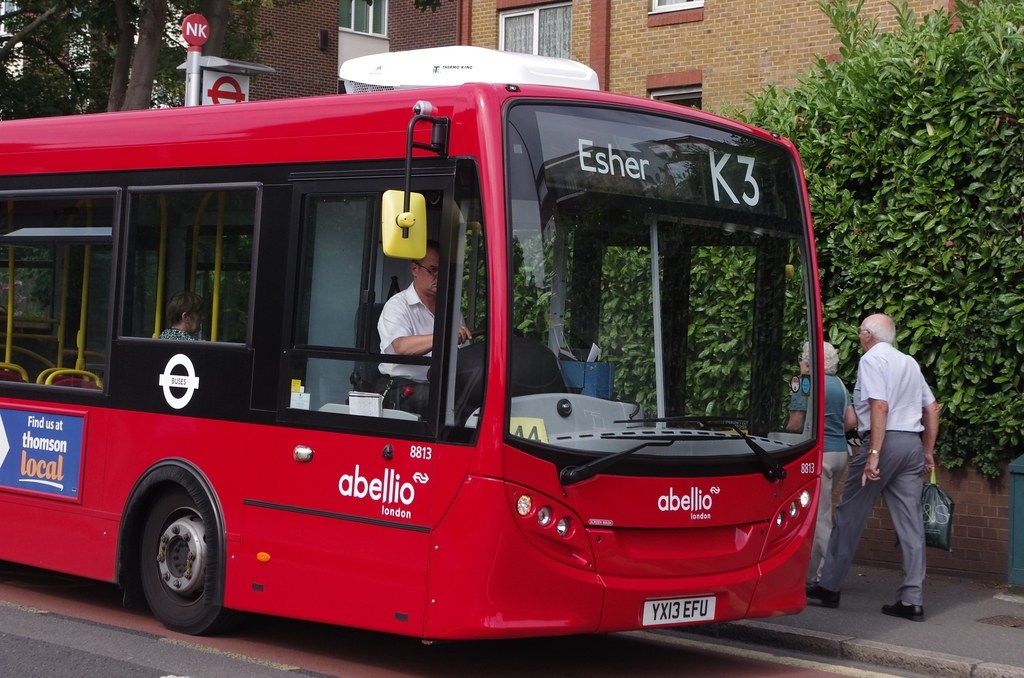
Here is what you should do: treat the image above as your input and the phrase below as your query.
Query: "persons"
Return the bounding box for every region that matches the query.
[374,240,473,421]
[806,312,940,621]
[157,288,207,341]
[785,340,858,584]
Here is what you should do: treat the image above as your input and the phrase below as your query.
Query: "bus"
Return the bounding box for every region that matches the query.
[0,44,825,647]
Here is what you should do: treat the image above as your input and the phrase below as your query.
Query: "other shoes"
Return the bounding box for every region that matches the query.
[805,575,819,585]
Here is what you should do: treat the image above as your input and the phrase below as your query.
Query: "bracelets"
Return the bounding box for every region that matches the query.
[868,449,879,454]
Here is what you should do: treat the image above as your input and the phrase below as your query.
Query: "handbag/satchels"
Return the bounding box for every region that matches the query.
[835,375,853,458]
[893,467,954,553]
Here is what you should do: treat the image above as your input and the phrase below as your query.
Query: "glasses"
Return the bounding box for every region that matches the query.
[857,328,869,337]
[415,262,439,276]
[797,353,810,364]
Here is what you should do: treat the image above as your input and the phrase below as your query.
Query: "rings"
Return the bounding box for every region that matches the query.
[871,473,873,476]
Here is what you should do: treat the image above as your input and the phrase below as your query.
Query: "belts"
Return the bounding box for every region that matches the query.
[862,429,916,439]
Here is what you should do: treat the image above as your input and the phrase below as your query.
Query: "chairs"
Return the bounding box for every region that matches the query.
[45,369,103,390]
[353,302,384,392]
[0,361,29,383]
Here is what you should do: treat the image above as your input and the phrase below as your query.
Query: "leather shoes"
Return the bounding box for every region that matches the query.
[804,583,841,608]
[881,600,925,622]
